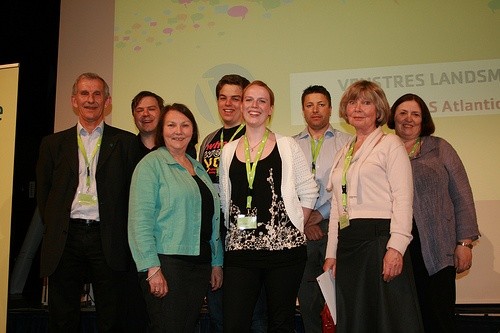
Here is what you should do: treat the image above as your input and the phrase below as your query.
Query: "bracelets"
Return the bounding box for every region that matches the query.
[455,241,476,251]
[145,266,161,280]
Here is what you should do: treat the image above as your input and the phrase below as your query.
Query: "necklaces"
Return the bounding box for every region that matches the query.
[244,136,264,151]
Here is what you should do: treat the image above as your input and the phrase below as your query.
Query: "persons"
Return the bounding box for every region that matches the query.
[385,93,482,333]
[289,85,353,332]
[35,73,146,333]
[126,103,223,333]
[198,73,249,333]
[131,91,197,158]
[218,80,317,333]
[322,80,414,333]
